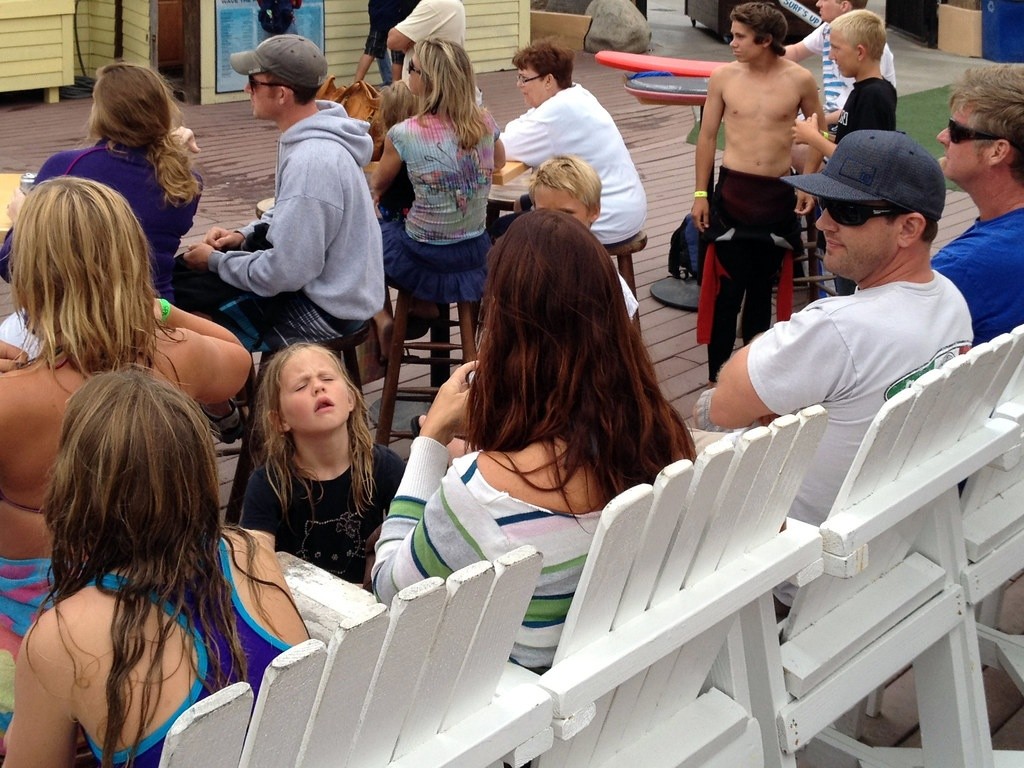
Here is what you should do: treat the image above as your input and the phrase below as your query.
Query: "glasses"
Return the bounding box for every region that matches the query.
[408,58,421,75]
[248,74,296,93]
[817,196,911,226]
[517,72,549,85]
[947,117,1024,154]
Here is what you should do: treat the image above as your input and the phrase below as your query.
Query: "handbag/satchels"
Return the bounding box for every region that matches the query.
[314,72,382,142]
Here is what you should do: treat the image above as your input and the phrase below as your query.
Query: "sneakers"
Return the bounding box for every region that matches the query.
[201,396,244,444]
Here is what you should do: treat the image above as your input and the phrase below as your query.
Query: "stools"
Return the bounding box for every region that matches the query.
[601,229,648,342]
[223,318,373,528]
[369,278,476,449]
[770,166,842,314]
[256,197,276,221]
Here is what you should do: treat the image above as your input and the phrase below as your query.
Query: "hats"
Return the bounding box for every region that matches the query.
[780,130,946,221]
[230,34,329,89]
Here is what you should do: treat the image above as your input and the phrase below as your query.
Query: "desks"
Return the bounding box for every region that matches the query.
[366,158,532,439]
[624,69,721,312]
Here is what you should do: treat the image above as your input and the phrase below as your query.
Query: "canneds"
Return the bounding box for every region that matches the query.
[20,172,36,195]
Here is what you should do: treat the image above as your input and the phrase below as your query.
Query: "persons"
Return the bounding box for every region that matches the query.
[241,344,407,586]
[690,0,899,389]
[1,364,309,767]
[0,62,206,307]
[371,210,695,680]
[170,0,645,454]
[685,128,974,619]
[929,60,1024,502]
[0,176,254,752]
[527,153,640,321]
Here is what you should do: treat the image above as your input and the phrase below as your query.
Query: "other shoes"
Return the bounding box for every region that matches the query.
[405,309,442,340]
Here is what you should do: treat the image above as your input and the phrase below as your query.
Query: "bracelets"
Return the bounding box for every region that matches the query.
[157,299,170,325]
[694,190,707,198]
[823,132,829,139]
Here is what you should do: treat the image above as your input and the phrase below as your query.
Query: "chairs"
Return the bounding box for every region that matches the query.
[159,325,1024,768]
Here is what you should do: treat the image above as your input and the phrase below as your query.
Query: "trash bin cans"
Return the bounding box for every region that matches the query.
[981,0,1024,63]
